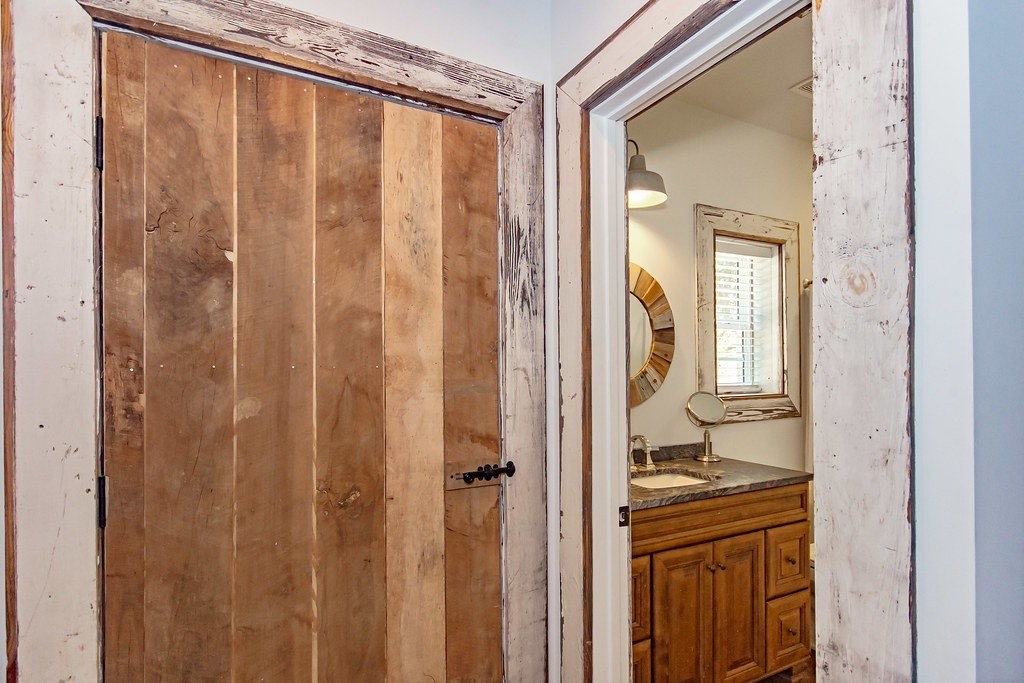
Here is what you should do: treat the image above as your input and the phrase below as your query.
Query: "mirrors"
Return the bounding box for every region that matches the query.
[630,266,675,409]
[685,392,728,462]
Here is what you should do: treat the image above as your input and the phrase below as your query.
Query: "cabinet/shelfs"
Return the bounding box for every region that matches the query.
[632,483,810,683]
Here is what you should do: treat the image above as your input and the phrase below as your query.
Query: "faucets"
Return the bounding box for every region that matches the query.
[628,436,651,470]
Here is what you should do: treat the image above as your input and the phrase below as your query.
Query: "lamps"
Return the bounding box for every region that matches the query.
[627,139,668,210]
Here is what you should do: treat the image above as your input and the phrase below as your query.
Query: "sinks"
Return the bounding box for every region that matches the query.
[630,468,721,490]
